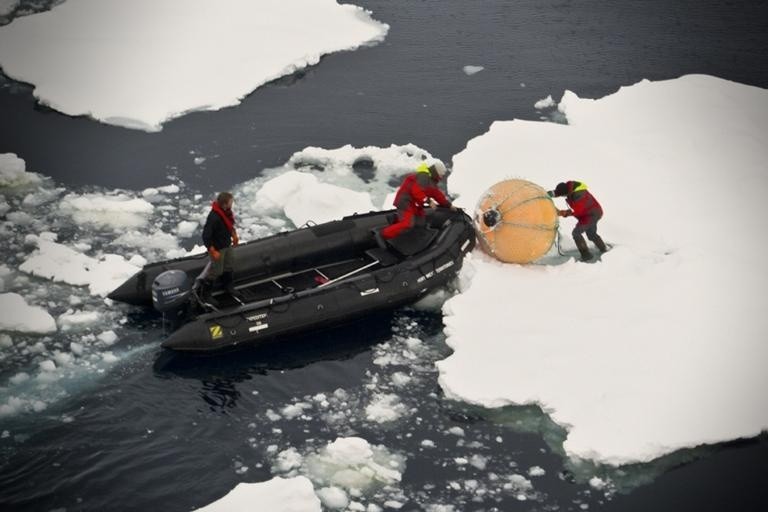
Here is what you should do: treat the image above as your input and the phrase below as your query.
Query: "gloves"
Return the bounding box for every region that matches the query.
[429,201,436,208]
[231,234,238,248]
[211,249,220,260]
[449,205,457,212]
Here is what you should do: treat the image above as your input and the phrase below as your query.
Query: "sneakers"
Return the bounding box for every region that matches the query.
[374,230,387,248]
[388,214,398,223]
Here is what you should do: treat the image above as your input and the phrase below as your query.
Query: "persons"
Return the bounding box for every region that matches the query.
[199,192,245,305]
[547,181,606,261]
[380,160,458,242]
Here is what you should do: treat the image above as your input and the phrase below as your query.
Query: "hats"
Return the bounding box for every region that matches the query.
[430,162,446,177]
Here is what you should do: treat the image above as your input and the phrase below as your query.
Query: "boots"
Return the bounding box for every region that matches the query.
[575,239,593,262]
[594,237,605,251]
[200,279,224,308]
[223,272,240,296]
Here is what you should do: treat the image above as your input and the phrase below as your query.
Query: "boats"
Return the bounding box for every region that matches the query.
[107,202,477,355]
